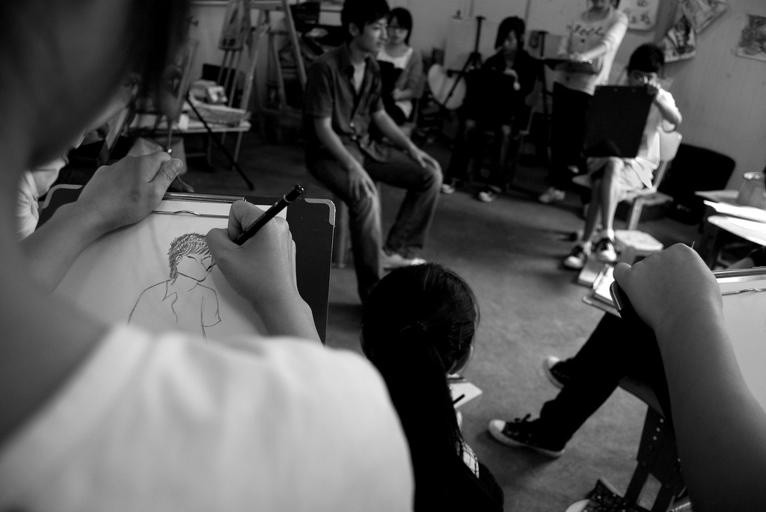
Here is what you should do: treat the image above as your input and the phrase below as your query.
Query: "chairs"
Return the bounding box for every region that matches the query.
[572,128,682,232]
[456,96,537,196]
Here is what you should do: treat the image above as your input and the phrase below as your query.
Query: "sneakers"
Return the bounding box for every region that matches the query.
[539,185,566,204]
[488,417,565,457]
[441,178,457,193]
[596,238,617,263]
[544,355,565,390]
[479,186,496,203]
[564,243,589,268]
[381,247,425,269]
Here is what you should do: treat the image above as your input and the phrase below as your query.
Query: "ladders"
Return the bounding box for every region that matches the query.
[243,0,307,144]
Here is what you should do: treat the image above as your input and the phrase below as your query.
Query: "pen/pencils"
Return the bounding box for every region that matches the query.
[205,184,303,273]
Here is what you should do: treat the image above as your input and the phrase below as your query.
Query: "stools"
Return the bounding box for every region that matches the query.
[306,181,384,272]
[612,225,662,267]
[584,375,694,512]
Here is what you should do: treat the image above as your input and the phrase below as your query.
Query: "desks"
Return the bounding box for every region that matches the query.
[699,199,765,271]
[121,117,252,171]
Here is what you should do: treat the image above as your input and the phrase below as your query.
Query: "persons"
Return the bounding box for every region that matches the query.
[1,2,505,511]
[440,1,683,269]
[489,243,766,511]
